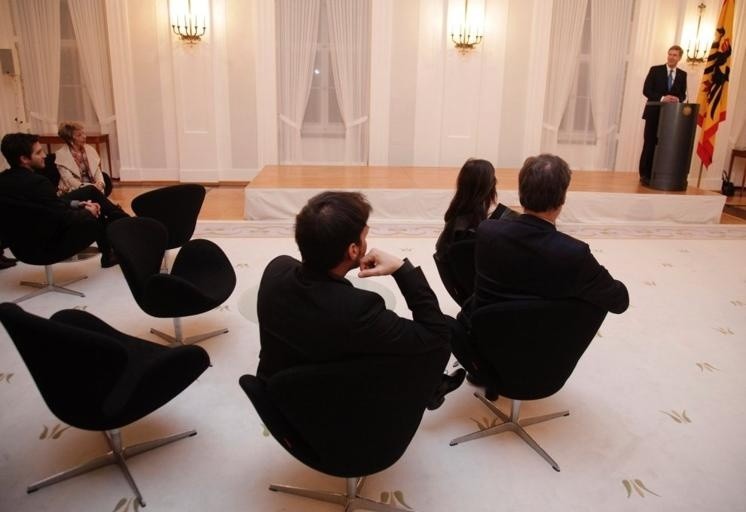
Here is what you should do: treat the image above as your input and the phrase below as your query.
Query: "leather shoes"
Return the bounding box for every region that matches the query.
[425,364,468,411]
[100,251,120,269]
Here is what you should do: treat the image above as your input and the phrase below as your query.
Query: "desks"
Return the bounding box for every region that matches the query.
[37,133,113,180]
[727,148,746,201]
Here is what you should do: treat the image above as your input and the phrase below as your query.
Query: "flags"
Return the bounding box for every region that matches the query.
[694,0,736,170]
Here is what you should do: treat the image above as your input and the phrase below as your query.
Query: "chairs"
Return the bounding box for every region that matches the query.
[50,169,115,200]
[104,215,239,350]
[0,199,91,305]
[0,298,212,511]
[122,182,208,273]
[232,370,435,512]
[445,292,610,470]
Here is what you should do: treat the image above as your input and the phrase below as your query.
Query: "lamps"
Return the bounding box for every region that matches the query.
[684,1,715,69]
[445,1,488,56]
[167,1,210,49]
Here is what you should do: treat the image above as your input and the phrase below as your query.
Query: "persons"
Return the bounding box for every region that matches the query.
[0,132,137,268]
[54,121,106,197]
[638,45,687,188]
[436,159,522,306]
[256,191,466,409]
[451,154,630,328]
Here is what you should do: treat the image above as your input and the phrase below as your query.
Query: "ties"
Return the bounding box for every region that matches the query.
[667,70,675,91]
